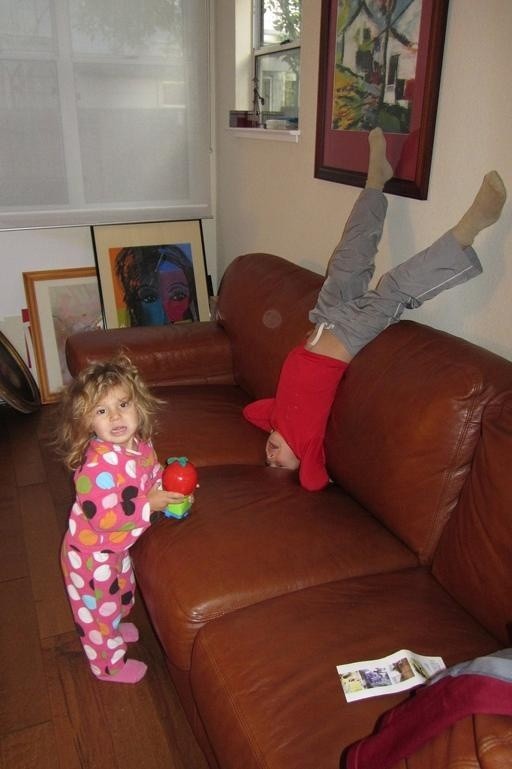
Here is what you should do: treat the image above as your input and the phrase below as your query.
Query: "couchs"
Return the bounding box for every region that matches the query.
[66,254,512,768]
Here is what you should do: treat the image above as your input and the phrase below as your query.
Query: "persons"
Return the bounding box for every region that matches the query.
[43,351,195,683]
[115,246,201,329]
[241,125,506,491]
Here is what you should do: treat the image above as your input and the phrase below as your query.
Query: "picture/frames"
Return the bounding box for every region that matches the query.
[89,219,213,330]
[23,268,105,404]
[314,0,448,200]
